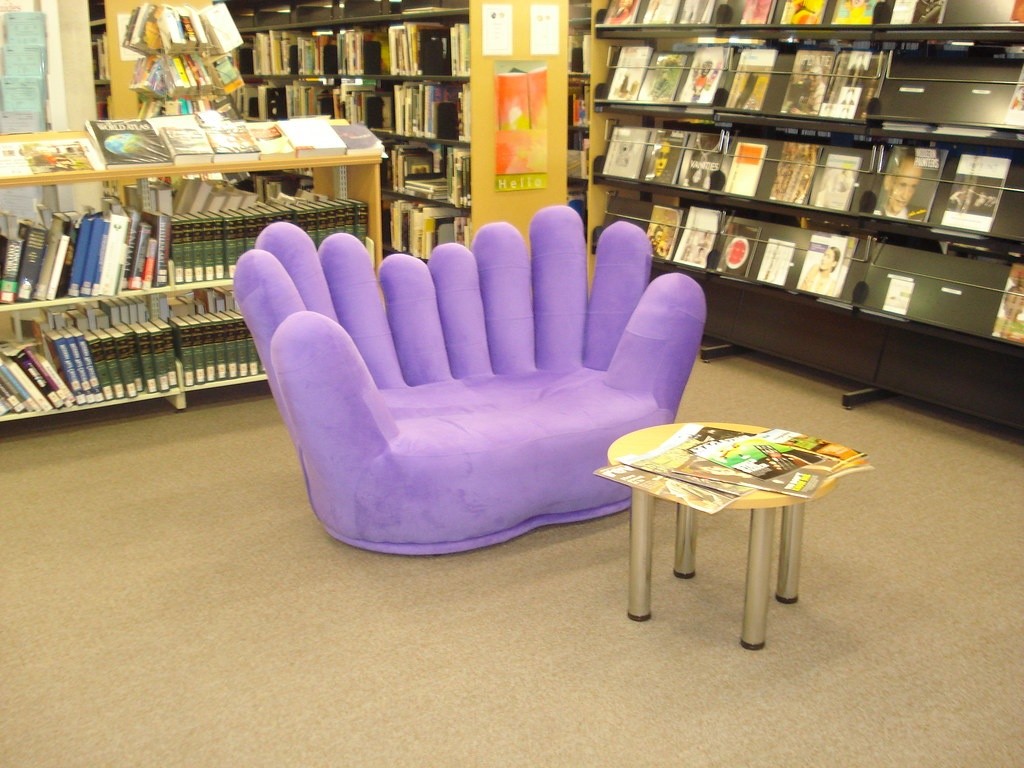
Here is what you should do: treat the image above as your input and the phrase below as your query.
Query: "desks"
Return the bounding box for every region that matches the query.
[608,421,873,651]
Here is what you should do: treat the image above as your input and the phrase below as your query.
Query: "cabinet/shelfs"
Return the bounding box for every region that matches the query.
[583,2,1024,441]
[1,124,391,431]
[226,0,571,257]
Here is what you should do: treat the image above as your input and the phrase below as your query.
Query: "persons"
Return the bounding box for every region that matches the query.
[874,148,926,221]
[800,247,841,292]
[824,55,865,118]
[786,54,827,115]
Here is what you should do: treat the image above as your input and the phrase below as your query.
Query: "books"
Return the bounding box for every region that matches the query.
[820,50,888,118]
[567,0,795,297]
[795,233,860,298]
[814,153,863,211]
[992,264,1024,344]
[593,423,875,515]
[890,1,947,24]
[872,145,949,221]
[780,0,829,24]
[1005,0,1024,126]
[831,0,878,24]
[769,142,824,204]
[0,4,470,416]
[940,154,1011,233]
[781,50,836,114]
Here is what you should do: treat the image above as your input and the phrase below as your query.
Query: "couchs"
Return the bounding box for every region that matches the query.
[232,206,711,554]
